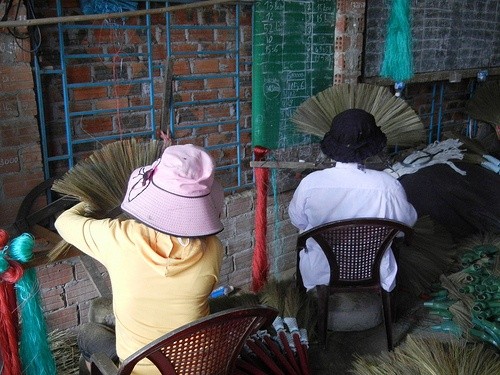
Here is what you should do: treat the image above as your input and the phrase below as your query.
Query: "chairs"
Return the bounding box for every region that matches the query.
[90,299,261,375]
[292,217,414,365]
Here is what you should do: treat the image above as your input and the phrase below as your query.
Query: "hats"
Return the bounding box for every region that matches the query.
[120,143,225,238]
[321,109,387,163]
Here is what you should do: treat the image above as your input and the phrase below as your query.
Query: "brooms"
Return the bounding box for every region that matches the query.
[289,82,429,163]
[47,137,173,262]
[44,276,499,375]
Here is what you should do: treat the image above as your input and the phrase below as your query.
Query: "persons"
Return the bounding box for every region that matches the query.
[53,143,224,375]
[289,108,417,293]
